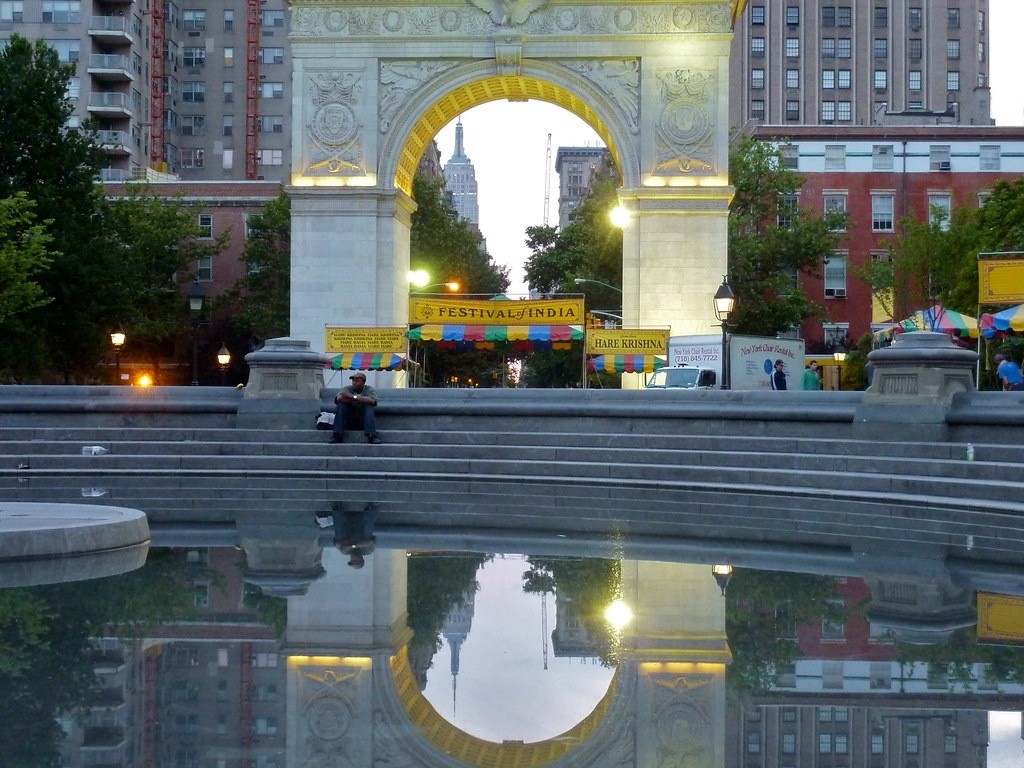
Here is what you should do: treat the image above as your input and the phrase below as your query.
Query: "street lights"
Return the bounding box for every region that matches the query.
[712,274,736,391]
[831,335,848,391]
[110,323,125,385]
[216,344,231,387]
[185,278,206,387]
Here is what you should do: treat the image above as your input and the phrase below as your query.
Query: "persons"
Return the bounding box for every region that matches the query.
[949,328,970,349]
[796,359,821,391]
[331,502,383,570]
[994,353,1024,391]
[770,358,787,391]
[327,371,382,445]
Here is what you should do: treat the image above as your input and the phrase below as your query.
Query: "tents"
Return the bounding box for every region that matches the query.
[404,291,587,396]
[324,347,423,389]
[582,344,667,387]
[869,302,1007,390]
[977,302,1024,388]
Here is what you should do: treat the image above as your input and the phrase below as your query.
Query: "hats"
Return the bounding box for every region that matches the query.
[349,372,366,380]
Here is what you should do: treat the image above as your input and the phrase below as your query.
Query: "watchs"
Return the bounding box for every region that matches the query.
[352,393,358,399]
[351,542,356,550]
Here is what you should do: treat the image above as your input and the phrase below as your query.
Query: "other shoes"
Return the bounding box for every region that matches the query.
[328,438,380,444]
